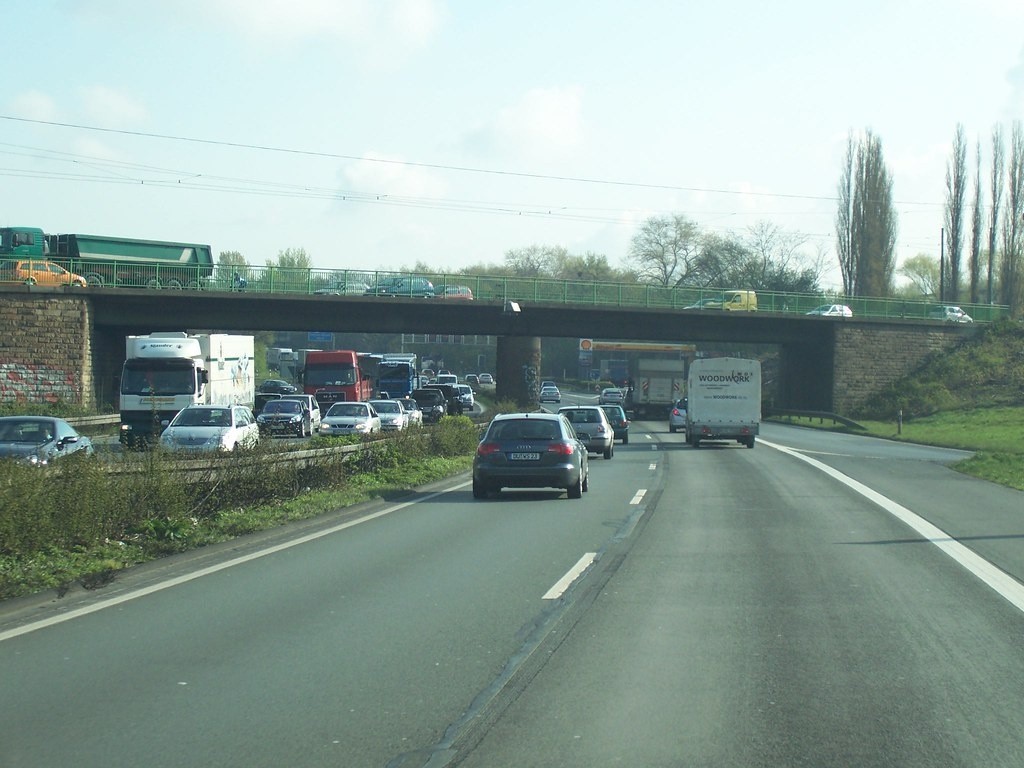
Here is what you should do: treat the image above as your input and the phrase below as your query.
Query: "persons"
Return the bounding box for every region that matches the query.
[358,408,365,415]
[39,423,53,441]
[234,271,247,291]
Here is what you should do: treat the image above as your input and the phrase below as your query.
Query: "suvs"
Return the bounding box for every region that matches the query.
[157,404,260,455]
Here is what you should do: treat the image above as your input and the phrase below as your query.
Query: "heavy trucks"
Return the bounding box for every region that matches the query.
[1,226,214,291]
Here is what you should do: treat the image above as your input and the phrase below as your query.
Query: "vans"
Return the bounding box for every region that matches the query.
[706,290,758,312]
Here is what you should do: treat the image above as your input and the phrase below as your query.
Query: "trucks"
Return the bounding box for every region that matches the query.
[687,357,762,451]
[303,349,376,408]
[631,358,686,419]
[267,348,417,416]
[115,331,256,448]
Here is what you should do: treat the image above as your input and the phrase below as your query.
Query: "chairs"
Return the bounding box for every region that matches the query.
[500,423,523,439]
[587,415,597,422]
[539,425,559,439]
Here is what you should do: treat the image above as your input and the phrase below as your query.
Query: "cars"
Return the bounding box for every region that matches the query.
[363,276,436,299]
[0,416,95,466]
[311,280,371,296]
[670,399,688,432]
[683,298,718,310]
[0,260,87,287]
[806,304,854,317]
[541,381,556,387]
[595,404,629,445]
[465,374,479,384]
[435,285,475,302]
[599,388,624,405]
[929,305,974,325]
[318,401,381,437]
[540,387,561,403]
[410,388,450,421]
[394,397,426,430]
[479,373,493,384]
[253,378,323,439]
[557,407,615,459]
[368,397,412,433]
[418,366,477,416]
[470,413,590,499]
